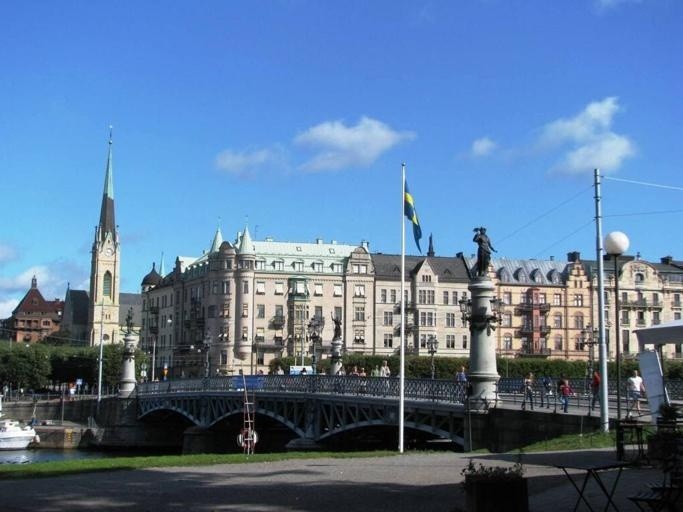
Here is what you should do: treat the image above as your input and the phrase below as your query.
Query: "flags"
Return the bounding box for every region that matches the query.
[403,177,423,255]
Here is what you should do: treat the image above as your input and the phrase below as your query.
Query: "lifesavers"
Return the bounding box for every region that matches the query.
[237,428,259,448]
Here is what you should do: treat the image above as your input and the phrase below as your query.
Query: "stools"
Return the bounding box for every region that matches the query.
[626,491,663,512]
[642,480,680,512]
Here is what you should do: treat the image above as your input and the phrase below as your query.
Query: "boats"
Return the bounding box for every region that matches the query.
[0,418,105,451]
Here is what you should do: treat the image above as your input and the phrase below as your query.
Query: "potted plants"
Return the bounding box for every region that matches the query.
[460,459,529,512]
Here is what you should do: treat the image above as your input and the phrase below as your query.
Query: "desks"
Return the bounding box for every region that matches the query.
[545,459,633,512]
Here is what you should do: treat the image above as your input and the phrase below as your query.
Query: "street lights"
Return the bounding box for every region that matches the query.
[504,335,511,378]
[604,230,630,461]
[255,334,259,375]
[578,322,600,395]
[310,333,320,375]
[167,314,177,380]
[203,333,213,378]
[427,334,438,379]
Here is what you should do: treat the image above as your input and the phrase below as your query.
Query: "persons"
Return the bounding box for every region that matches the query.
[472,226,497,277]
[556,372,566,410]
[520,371,537,410]
[68,385,76,401]
[626,369,646,418]
[455,366,468,403]
[330,311,342,340]
[558,379,576,413]
[539,370,551,410]
[212,359,390,397]
[590,370,601,412]
